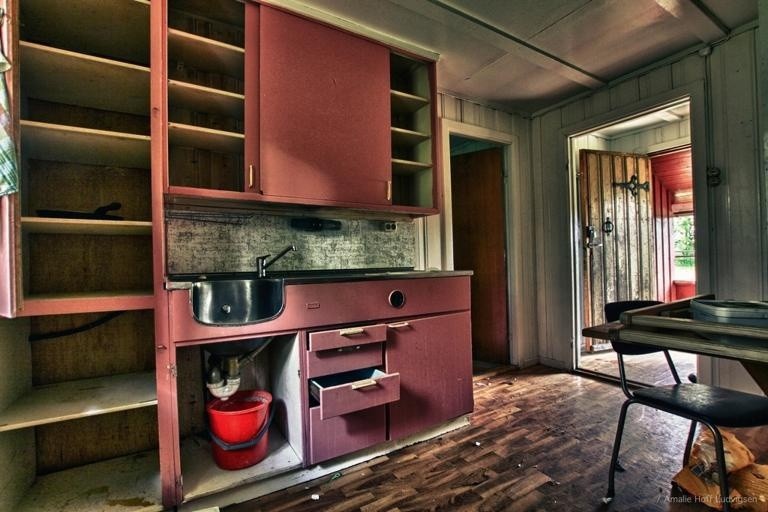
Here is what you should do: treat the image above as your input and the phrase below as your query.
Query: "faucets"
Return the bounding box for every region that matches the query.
[257,245,297,277]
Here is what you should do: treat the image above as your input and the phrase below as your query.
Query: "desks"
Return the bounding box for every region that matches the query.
[582,293,768,401]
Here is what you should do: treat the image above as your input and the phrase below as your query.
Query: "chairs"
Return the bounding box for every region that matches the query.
[602,299,768,510]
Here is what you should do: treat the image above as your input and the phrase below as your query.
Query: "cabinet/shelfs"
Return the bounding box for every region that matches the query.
[386,309,475,444]
[162,0,444,220]
[1,0,175,512]
[299,319,401,466]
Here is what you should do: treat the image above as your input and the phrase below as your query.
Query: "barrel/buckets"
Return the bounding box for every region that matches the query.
[207,389,275,468]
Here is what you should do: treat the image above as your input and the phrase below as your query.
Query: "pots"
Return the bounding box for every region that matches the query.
[33,202,130,221]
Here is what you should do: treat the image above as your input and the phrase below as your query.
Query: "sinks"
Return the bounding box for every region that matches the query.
[188,279,284,326]
